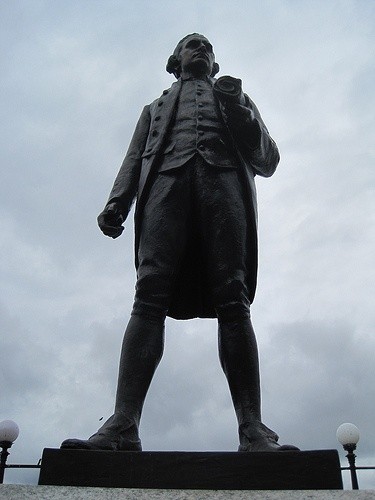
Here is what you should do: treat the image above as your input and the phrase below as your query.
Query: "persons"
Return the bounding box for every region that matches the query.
[59,31,305,451]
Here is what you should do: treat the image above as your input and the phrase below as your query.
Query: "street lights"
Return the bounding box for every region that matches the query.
[0,420,19,484]
[336,423,360,490]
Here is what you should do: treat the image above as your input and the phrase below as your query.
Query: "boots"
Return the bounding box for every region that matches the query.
[218,315,298,452]
[61,315,166,449]
